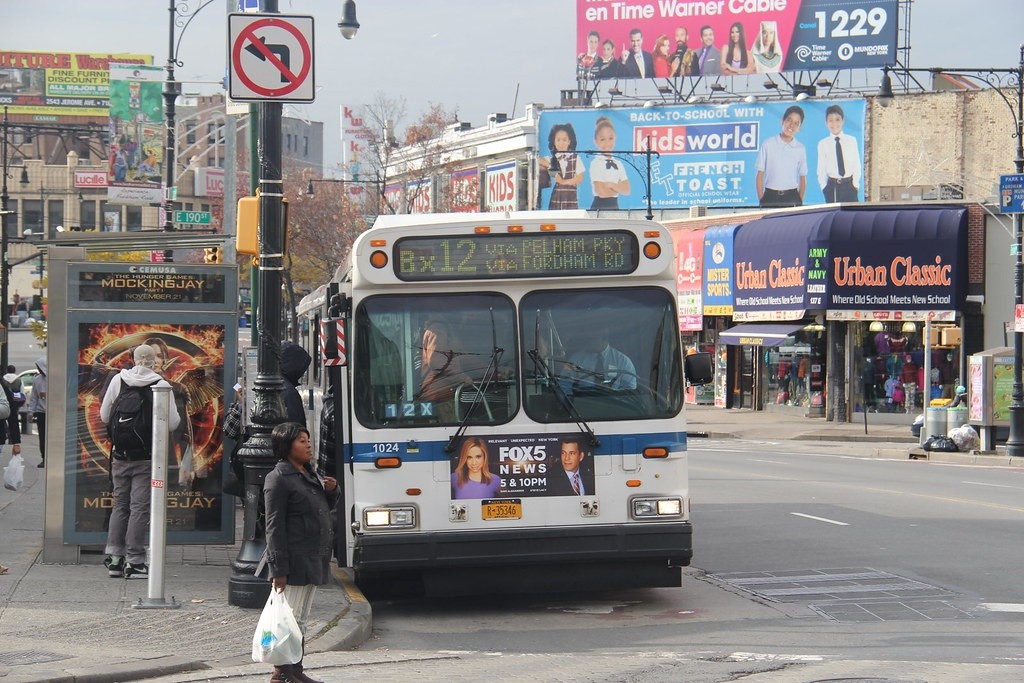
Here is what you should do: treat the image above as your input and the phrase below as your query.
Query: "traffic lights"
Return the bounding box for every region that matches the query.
[215,246,226,265]
[203,246,217,266]
[235,196,290,262]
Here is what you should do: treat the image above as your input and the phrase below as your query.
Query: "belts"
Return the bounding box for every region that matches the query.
[765,187,797,195]
[829,177,852,184]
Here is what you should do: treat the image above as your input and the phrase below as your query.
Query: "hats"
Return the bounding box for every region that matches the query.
[956,386,966,393]
[134,345,154,361]
[906,354,912,360]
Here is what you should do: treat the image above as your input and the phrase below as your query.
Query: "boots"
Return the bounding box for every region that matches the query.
[270,636,325,683]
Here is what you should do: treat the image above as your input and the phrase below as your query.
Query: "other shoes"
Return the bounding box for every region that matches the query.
[0,565,9,575]
[37,461,44,468]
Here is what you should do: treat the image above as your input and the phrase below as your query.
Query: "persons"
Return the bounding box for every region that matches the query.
[798,355,808,393]
[588,116,629,210]
[0,357,47,468]
[753,106,807,208]
[421,305,634,425]
[778,357,791,406]
[451,437,507,499]
[577,21,782,80]
[282,338,312,429]
[111,145,127,181]
[142,339,186,451]
[254,422,341,683]
[862,356,878,413]
[547,124,584,210]
[100,347,181,579]
[547,438,595,496]
[134,151,155,181]
[938,353,955,401]
[816,104,860,205]
[900,357,919,414]
[224,377,246,506]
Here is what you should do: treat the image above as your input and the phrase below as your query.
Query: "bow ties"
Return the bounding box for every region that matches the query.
[557,154,569,161]
[606,159,619,170]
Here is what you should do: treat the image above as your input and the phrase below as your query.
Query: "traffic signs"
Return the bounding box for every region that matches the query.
[174,210,211,225]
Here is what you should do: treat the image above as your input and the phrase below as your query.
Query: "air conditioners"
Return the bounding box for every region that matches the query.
[690,205,708,218]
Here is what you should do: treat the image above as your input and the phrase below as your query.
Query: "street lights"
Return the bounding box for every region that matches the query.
[163,0,360,262]
[875,45,1024,457]
[548,134,660,220]
[0,106,30,371]
[38,181,84,296]
[307,169,384,215]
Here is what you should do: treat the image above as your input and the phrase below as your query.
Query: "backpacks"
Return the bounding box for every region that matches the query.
[108,376,162,461]
[412,320,451,389]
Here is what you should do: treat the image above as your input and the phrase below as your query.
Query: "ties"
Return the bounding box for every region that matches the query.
[835,137,845,176]
[595,354,605,383]
[572,474,581,496]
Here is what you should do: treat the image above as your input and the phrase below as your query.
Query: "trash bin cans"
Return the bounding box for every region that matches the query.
[927,408,946,438]
[945,408,968,434]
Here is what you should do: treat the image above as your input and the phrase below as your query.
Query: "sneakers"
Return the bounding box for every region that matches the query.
[104,556,149,579]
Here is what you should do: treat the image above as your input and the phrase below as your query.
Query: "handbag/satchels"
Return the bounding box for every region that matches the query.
[3,453,25,491]
[228,434,249,480]
[9,391,26,406]
[252,585,303,665]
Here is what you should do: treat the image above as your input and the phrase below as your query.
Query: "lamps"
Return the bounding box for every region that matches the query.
[901,321,917,332]
[763,79,793,94]
[16,303,29,328]
[30,294,43,322]
[869,321,883,332]
[711,83,747,98]
[815,79,853,93]
[608,89,649,102]
[657,86,693,96]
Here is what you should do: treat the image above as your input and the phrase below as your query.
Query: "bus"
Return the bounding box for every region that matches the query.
[297,210,714,604]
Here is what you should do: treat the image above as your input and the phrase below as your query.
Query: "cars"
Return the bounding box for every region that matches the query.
[17,369,40,413]
